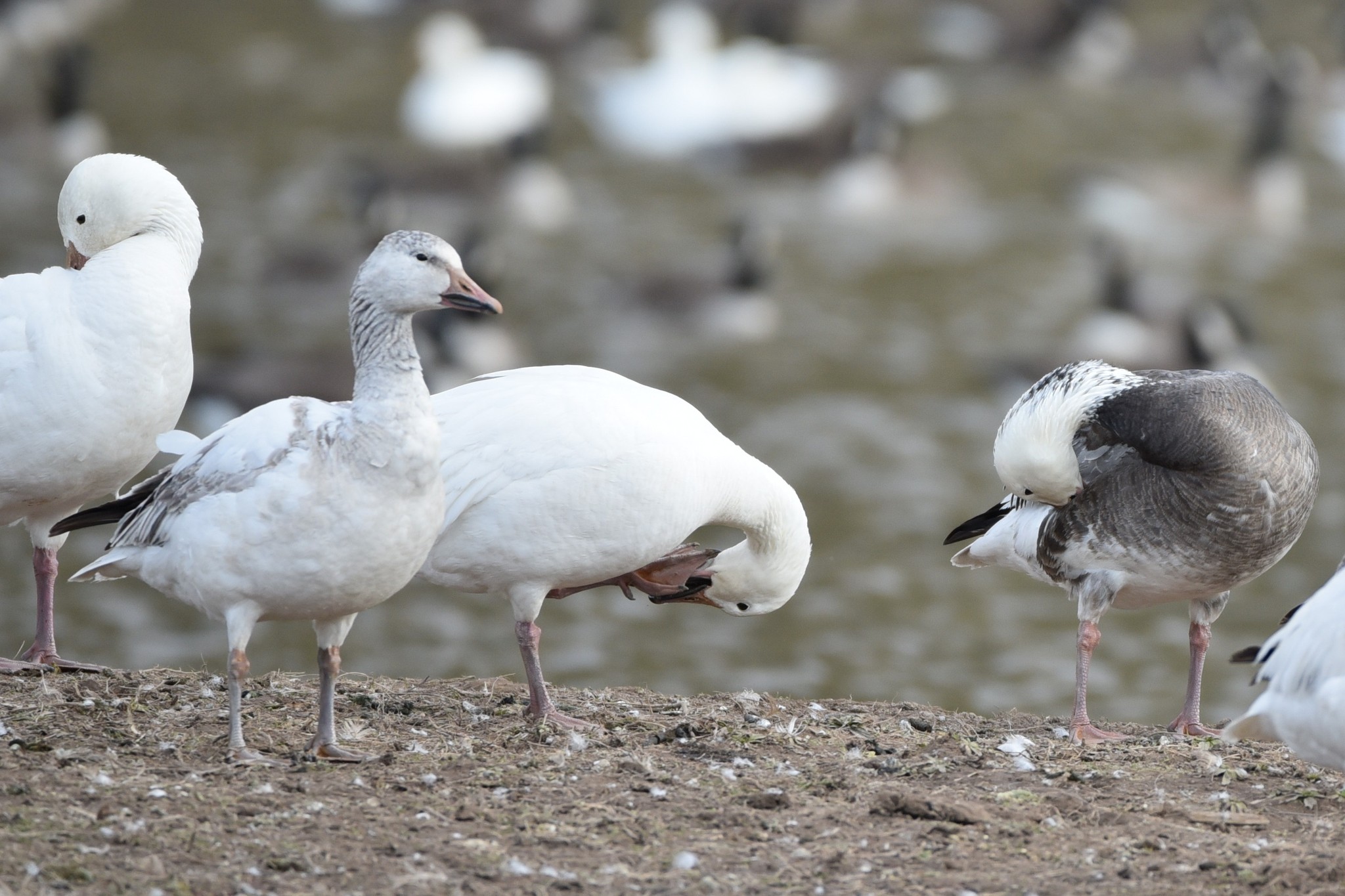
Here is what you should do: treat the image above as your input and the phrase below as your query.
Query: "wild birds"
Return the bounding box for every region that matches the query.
[1224,567,1345,772]
[432,365,815,745]
[1,151,203,675]
[43,227,501,770]
[0,3,1345,392]
[940,354,1320,743]
